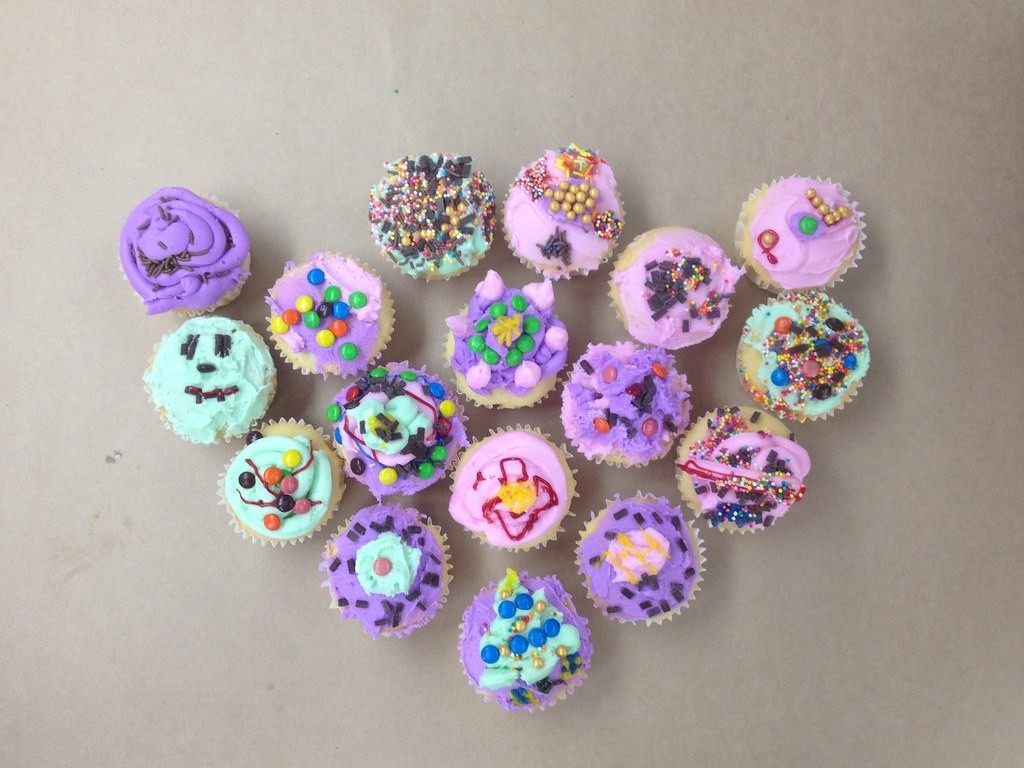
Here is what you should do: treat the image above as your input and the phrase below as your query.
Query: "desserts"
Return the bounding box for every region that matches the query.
[115,135,872,714]
